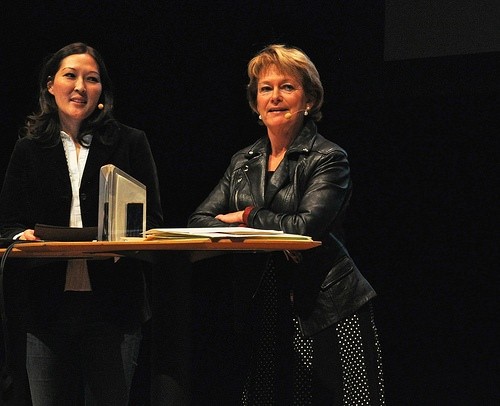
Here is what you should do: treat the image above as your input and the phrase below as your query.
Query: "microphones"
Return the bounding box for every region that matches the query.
[285,109,304,119]
[98,102,103,109]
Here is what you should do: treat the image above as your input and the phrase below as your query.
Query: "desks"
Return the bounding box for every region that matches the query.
[0,238,322,406]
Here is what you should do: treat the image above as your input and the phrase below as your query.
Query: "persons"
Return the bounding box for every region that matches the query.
[187,44,385,406]
[0,43,165,406]
